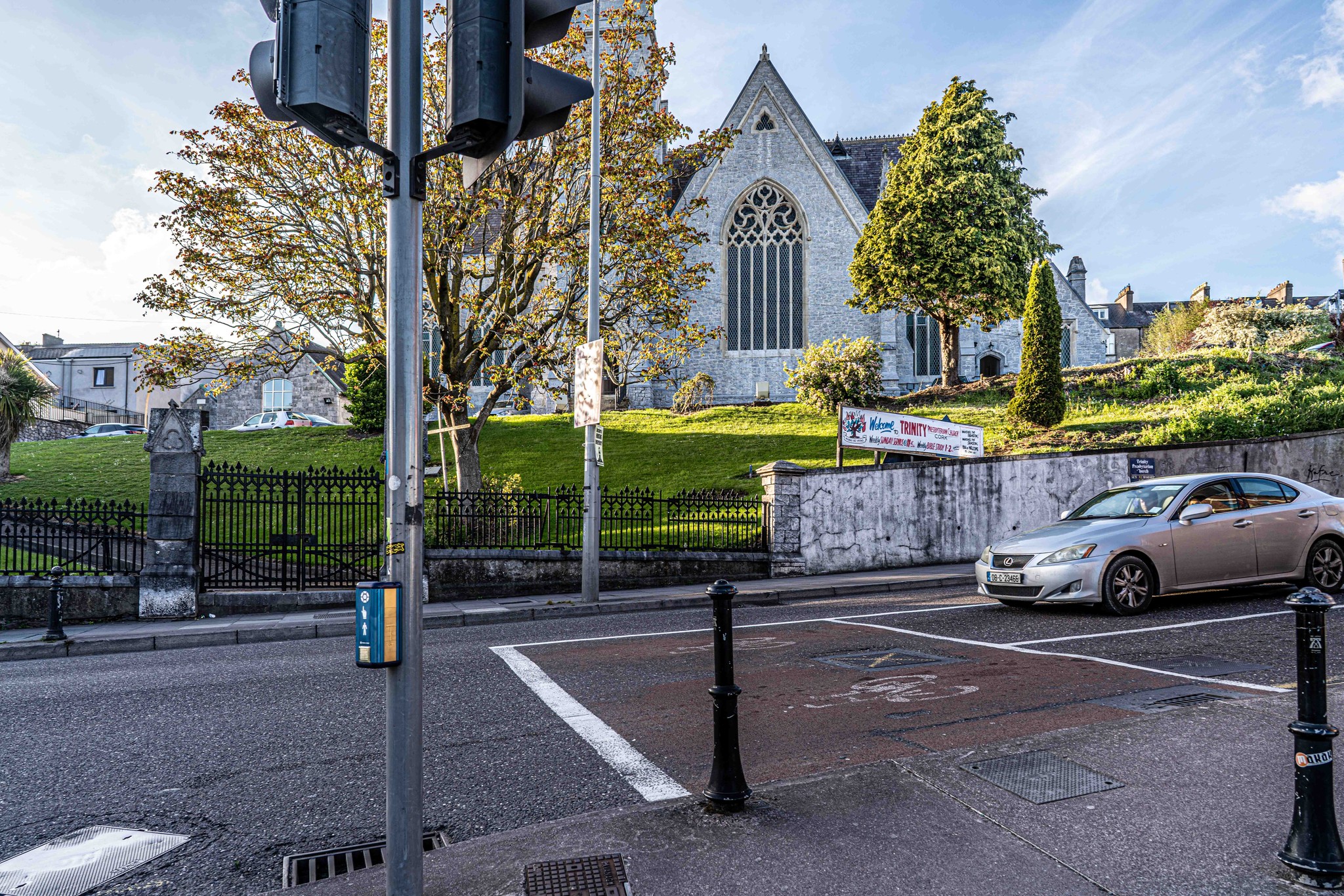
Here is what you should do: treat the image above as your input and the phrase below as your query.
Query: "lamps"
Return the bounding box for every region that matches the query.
[754,381,770,402]
[1329,298,1336,305]
[988,341,994,351]
[323,397,332,404]
[556,395,570,412]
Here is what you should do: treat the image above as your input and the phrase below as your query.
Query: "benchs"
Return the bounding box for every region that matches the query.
[1244,490,1295,507]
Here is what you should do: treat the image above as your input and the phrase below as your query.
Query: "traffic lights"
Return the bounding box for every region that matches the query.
[247,0,374,149]
[446,0,595,158]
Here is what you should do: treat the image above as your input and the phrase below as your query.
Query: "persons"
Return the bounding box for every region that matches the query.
[1187,495,1207,506]
[1140,491,1158,513]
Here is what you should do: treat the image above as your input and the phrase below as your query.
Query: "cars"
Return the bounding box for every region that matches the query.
[974,471,1344,617]
[64,423,149,440]
[228,411,312,431]
[472,400,530,416]
[305,414,348,428]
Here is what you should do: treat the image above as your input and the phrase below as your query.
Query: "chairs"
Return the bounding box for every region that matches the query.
[1159,495,1179,521]
[498,402,506,408]
[101,428,104,433]
[105,426,110,432]
[263,418,269,423]
